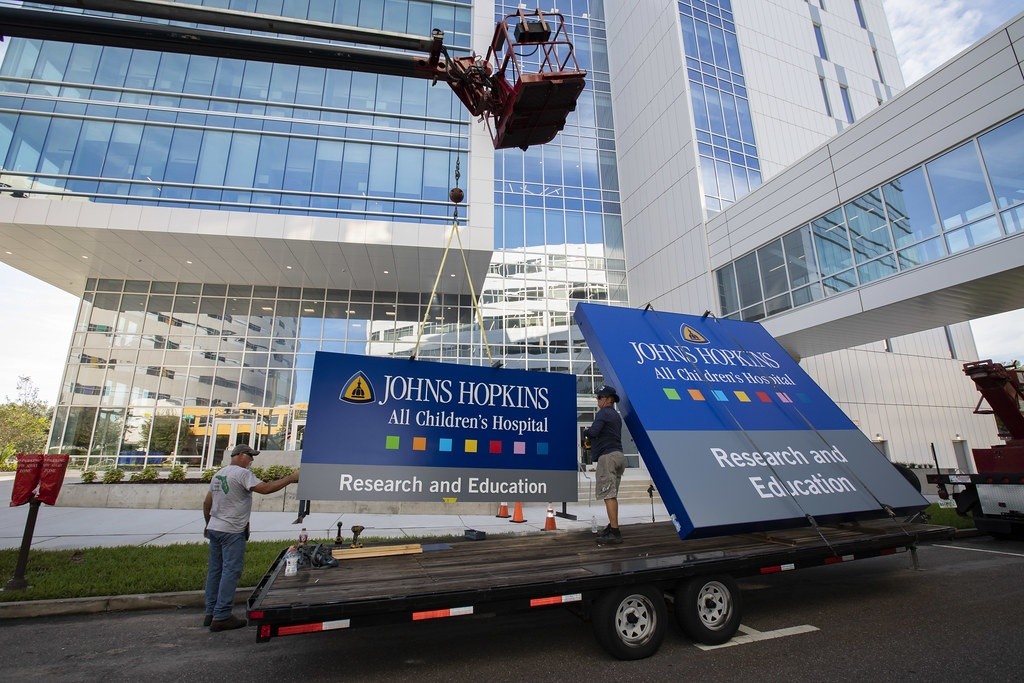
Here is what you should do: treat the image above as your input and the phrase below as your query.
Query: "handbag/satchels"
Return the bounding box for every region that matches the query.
[244,522,250,541]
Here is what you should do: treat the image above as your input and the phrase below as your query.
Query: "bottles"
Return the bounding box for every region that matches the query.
[299,527,308,545]
[591,515,598,533]
[285,548,298,576]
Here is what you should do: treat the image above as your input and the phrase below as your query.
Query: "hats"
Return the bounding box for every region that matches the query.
[596,386,620,403]
[230,444,260,457]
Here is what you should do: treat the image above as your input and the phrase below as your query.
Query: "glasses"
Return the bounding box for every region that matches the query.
[596,396,607,400]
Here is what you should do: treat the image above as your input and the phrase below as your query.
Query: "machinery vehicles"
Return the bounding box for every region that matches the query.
[2,0,591,227]
[925,359,1024,531]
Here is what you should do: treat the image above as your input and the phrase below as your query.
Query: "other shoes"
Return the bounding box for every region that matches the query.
[203,615,214,626]
[209,614,247,632]
[594,523,623,545]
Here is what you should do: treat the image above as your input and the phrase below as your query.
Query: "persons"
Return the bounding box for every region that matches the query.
[584,385,627,545]
[203,444,299,632]
